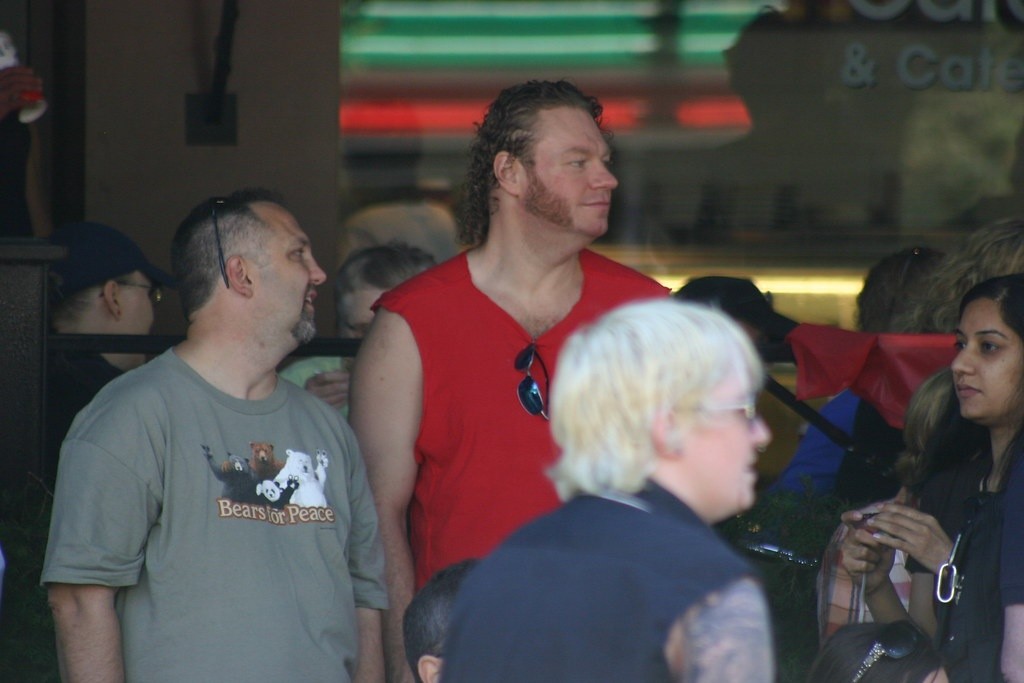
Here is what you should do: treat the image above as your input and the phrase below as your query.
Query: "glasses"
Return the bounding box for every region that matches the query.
[202,196,230,289]
[100,279,163,307]
[851,619,932,683]
[514,342,551,422]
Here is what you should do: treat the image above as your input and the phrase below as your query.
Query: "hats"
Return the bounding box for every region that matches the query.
[46,221,153,303]
[675,275,800,360]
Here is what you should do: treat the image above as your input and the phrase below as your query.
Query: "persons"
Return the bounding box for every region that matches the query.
[345,81,671,683]
[42,191,389,683]
[402,557,480,683]
[437,298,772,683]
[300,243,437,411]
[775,217,1024,683]
[45,220,174,494]
